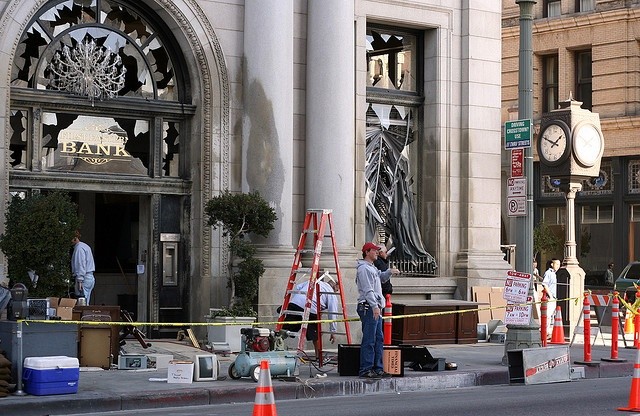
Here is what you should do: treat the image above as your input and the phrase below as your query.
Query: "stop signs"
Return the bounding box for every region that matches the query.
[507,177,528,216]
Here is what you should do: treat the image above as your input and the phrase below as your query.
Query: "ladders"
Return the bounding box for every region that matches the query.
[276,208,350,367]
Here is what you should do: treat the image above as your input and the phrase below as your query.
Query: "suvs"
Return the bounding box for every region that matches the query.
[614,262,640,291]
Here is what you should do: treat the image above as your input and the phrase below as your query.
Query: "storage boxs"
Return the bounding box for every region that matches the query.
[23,356,80,396]
[167,360,194,383]
[45,297,77,321]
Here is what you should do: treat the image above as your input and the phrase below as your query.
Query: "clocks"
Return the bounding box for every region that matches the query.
[570,119,606,168]
[536,120,571,166]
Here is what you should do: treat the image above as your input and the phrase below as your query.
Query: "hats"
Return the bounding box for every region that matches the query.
[362,243,381,252]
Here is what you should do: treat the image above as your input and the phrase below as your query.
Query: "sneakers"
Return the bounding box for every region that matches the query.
[359,368,380,379]
[372,368,392,378]
[315,357,332,366]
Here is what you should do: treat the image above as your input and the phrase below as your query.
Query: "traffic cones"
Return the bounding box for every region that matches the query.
[253,360,276,416]
[617,343,640,411]
[547,306,569,344]
[623,303,633,334]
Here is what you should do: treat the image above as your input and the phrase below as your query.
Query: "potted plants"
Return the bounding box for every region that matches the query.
[203,189,278,354]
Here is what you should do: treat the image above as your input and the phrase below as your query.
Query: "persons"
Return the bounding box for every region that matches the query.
[375,245,393,300]
[70,235,96,306]
[278,277,342,365]
[531,260,541,292]
[604,262,615,287]
[542,258,561,341]
[354,243,401,383]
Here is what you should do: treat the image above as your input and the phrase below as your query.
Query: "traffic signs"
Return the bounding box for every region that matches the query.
[504,119,531,150]
[505,296,532,325]
[512,149,524,178]
[503,271,531,303]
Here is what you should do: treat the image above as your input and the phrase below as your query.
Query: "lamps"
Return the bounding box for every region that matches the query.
[49,36,128,108]
